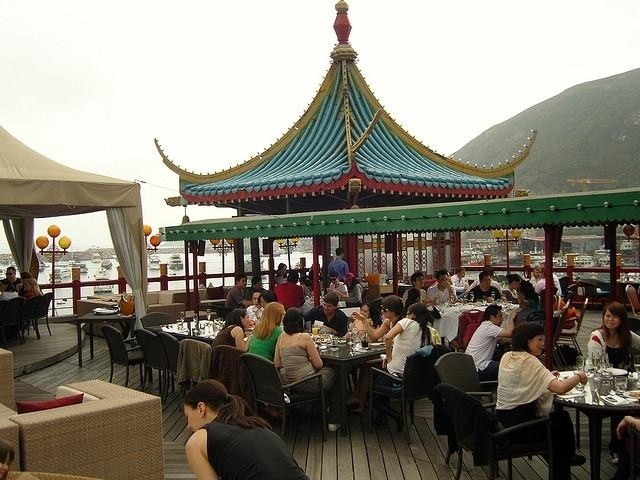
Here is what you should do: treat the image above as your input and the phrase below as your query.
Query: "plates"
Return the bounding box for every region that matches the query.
[622,389,640,399]
[609,368,628,376]
[93,307,118,316]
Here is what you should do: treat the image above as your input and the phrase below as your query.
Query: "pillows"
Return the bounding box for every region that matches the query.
[16,393,84,414]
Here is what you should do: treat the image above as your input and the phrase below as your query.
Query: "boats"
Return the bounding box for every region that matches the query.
[0,250,184,303]
[531,249,624,270]
[259,247,294,258]
[243,255,267,281]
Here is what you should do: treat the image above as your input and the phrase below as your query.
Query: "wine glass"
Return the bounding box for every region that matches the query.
[176,308,224,336]
[572,351,612,397]
[311,317,369,355]
[426,291,515,313]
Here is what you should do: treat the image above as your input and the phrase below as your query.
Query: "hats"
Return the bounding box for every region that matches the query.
[323,293,339,306]
[344,273,355,281]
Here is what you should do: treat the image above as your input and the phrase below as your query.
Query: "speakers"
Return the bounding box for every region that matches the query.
[552,225,563,253]
[385,235,394,253]
[198,240,206,256]
[263,239,272,254]
[604,225,612,250]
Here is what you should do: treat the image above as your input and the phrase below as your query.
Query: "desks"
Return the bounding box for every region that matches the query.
[77,312,136,368]
[150,318,398,437]
[536,364,638,478]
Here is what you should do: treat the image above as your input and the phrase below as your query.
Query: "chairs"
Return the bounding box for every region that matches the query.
[133,327,163,396]
[431,353,501,476]
[622,286,640,327]
[0,292,54,348]
[366,344,448,445]
[0,379,164,480]
[139,310,170,327]
[210,345,251,419]
[417,286,548,355]
[175,337,212,402]
[550,298,571,349]
[558,298,592,353]
[104,324,149,388]
[155,330,186,402]
[433,379,562,478]
[238,351,333,445]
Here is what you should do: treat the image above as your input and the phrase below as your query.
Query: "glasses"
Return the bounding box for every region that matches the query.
[329,279,336,282]
[380,305,389,313]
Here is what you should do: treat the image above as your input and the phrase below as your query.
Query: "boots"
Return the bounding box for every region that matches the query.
[366,394,403,432]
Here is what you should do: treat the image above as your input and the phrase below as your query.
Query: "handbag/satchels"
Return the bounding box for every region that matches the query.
[554,345,576,366]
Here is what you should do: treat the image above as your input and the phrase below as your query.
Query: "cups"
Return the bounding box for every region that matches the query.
[613,376,627,394]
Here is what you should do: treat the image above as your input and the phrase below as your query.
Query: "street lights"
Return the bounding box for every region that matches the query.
[493,227,521,275]
[277,237,299,270]
[142,225,158,253]
[36,225,71,319]
[211,240,234,288]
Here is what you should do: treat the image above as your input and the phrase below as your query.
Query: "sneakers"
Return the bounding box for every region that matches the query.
[570,453,586,466]
[328,423,341,432]
[609,450,619,464]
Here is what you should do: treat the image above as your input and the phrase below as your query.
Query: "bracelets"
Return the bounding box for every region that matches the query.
[576,373,582,382]
[630,420,638,430]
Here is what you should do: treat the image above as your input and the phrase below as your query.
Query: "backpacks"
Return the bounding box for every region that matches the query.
[463,311,485,344]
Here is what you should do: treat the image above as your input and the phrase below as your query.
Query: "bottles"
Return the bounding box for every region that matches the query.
[116,295,134,316]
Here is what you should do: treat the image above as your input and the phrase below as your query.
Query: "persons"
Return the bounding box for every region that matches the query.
[16,271,45,304]
[182,380,311,480]
[325,246,349,277]
[587,301,639,462]
[0,439,15,480]
[213,262,561,432]
[616,415,640,440]
[495,321,587,467]
[0,267,23,292]
